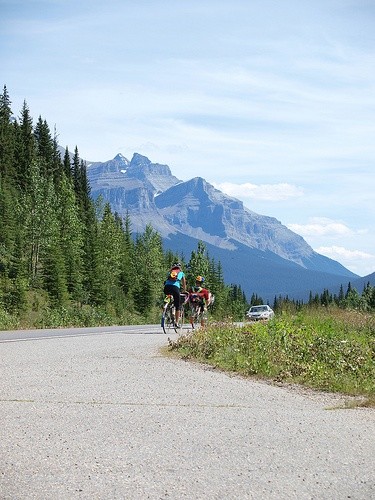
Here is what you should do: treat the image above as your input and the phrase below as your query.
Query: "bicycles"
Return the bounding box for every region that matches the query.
[190,301,212,329]
[160,292,188,334]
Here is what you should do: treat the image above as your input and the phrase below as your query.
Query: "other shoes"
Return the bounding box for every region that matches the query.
[175,322,180,327]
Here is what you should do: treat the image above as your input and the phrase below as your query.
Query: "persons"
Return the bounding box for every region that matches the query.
[188,276,214,326]
[163,267,186,328]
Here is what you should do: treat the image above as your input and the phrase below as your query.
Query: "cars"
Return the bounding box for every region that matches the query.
[245,305,275,321]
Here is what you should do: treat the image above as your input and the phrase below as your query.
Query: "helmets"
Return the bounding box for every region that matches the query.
[196,276,205,283]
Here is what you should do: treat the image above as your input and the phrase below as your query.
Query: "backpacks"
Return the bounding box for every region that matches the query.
[167,269,181,282]
[190,286,202,303]
